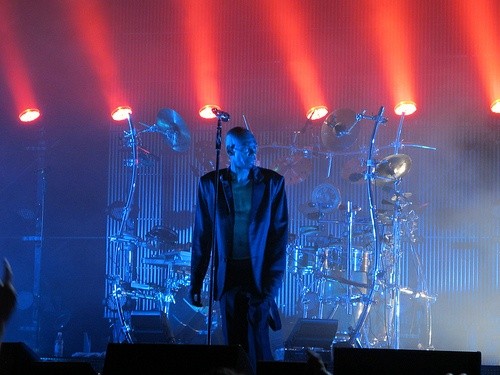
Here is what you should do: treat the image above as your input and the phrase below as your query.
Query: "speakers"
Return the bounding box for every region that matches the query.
[333,347,482,375]
[0,343,97,375]
[98,341,256,375]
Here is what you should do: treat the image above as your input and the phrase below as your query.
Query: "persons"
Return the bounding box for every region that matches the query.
[189,125,290,368]
[0,256,19,342]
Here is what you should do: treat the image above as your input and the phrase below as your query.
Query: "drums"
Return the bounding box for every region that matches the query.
[290,244,370,274]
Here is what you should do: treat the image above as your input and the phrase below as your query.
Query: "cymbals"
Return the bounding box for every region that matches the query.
[157,108,192,151]
[321,108,360,150]
[370,153,412,185]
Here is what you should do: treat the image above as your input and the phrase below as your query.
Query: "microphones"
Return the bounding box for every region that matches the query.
[348,170,367,182]
[212,108,230,122]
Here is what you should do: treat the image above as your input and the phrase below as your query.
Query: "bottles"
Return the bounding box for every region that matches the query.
[55,332,63,358]
[83,332,91,353]
